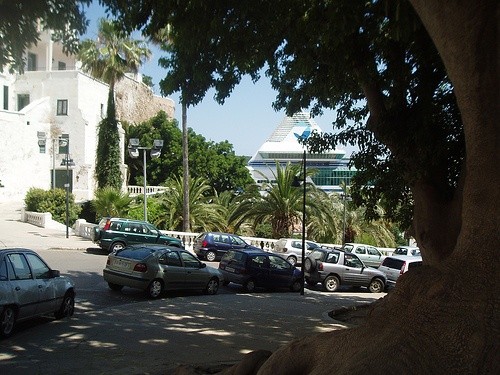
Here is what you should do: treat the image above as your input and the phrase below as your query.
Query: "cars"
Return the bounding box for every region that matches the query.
[272,238,388,266]
[193,231,260,262]
[103,243,224,298]
[0,248,77,339]
[218,248,302,293]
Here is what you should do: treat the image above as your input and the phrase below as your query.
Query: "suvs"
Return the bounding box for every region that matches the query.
[91,217,185,252]
[304,246,388,293]
[377,245,423,293]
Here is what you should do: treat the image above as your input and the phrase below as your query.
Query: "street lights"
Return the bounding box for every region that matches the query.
[128,138,164,221]
[60,151,76,238]
[290,150,307,295]
[37,130,68,189]
